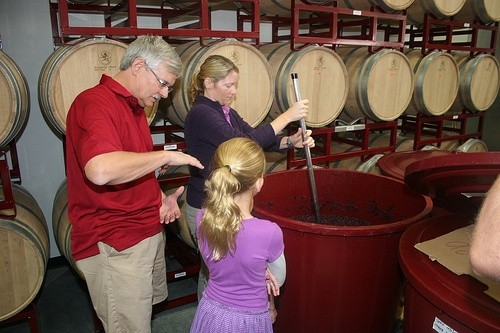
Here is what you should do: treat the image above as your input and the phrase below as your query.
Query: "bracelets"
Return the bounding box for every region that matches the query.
[286,135,293,148]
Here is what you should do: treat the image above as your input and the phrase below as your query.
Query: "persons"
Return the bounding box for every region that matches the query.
[64,35,204,333]
[468,175,500,279]
[184,55,315,303]
[190,137,286,333]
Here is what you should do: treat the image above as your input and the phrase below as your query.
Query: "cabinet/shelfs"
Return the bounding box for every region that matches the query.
[0,0,498,333]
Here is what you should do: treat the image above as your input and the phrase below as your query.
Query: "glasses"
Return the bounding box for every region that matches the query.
[144,59,174,94]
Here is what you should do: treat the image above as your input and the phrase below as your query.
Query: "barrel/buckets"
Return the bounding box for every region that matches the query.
[255,41,350,130]
[397,47,461,117]
[234,0,333,19]
[445,51,500,112]
[402,0,466,26]
[338,0,416,14]
[52,176,86,283]
[258,132,500,333]
[453,0,500,25]
[0,182,50,322]
[334,45,415,125]
[165,38,276,133]
[156,165,201,250]
[0,49,30,149]
[38,36,159,137]
